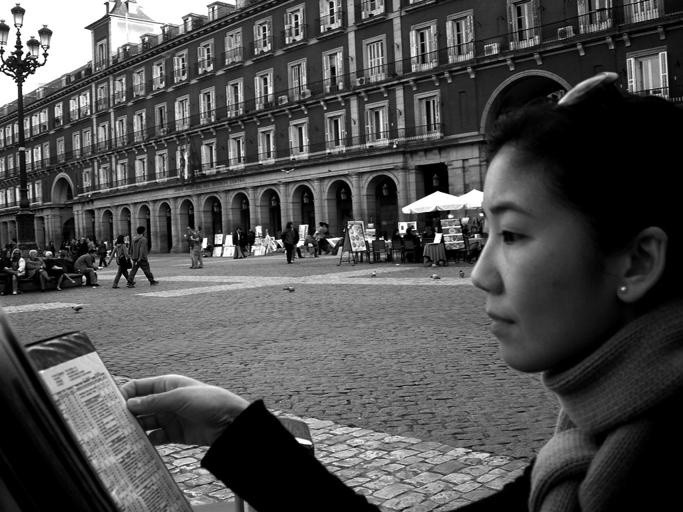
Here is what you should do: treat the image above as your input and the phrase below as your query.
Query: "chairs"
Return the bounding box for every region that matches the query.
[352,237,448,267]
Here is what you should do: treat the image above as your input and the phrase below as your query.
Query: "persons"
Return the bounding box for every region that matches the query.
[116,85,682,510]
[108,236,135,288]
[230,220,433,264]
[126,226,158,287]
[0,234,107,295]
[184,225,204,269]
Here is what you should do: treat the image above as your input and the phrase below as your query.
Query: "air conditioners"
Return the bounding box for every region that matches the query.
[482,42,499,57]
[276,95,288,107]
[299,88,311,101]
[354,77,368,86]
[557,25,575,42]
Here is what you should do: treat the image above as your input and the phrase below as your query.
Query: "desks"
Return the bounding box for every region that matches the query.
[464,237,486,263]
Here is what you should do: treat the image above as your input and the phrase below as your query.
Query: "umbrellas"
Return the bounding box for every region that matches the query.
[457,188,483,210]
[401,189,465,214]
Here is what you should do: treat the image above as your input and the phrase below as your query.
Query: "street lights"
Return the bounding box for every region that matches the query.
[0,2,52,253]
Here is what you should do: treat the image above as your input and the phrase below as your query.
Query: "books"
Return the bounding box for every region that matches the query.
[0,311,194,512]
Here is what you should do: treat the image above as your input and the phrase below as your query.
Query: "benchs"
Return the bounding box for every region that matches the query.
[0,272,84,295]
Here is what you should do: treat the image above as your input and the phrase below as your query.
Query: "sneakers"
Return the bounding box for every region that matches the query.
[150,280,159,286]
[112,285,120,289]
[126,281,136,288]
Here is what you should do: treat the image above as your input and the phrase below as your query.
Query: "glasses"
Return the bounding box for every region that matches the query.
[552,69,633,119]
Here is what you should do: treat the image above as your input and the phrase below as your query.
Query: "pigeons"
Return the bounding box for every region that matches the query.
[430,273,440,279]
[283,286,296,293]
[371,270,377,277]
[459,269,465,278]
[71,306,83,312]
